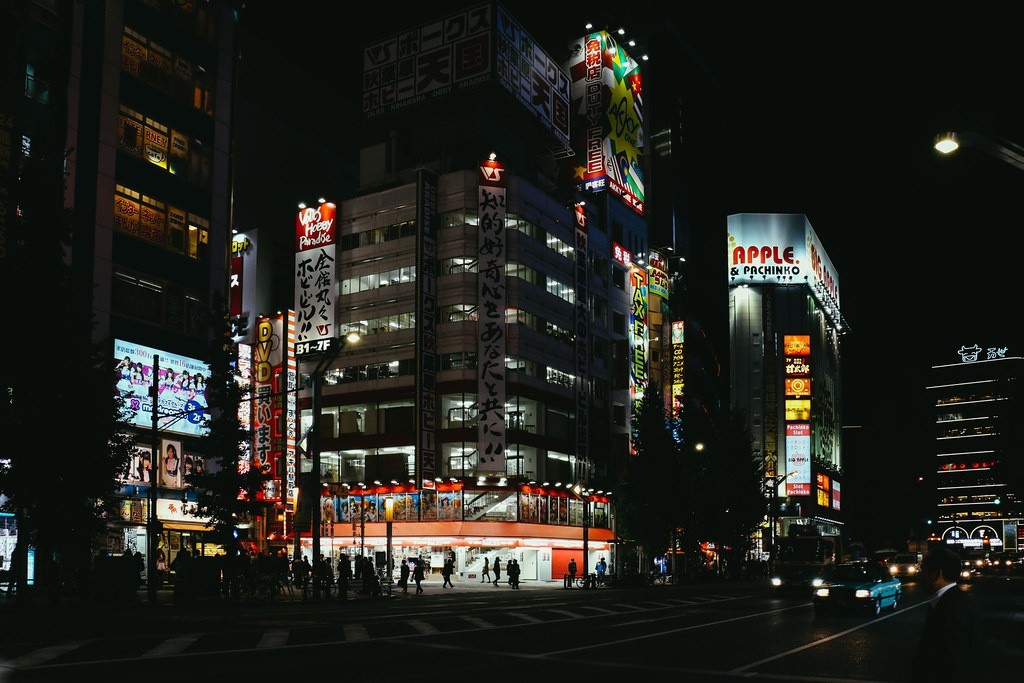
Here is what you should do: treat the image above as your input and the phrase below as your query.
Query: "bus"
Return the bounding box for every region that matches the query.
[768,535,835,600]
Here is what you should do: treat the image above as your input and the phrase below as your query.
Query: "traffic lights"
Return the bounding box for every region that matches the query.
[208,361,230,411]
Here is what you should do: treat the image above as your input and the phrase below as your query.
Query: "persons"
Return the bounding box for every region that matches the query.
[184,458,204,478]
[480,557,520,590]
[114,355,162,397]
[441,549,456,588]
[294,553,375,602]
[133,450,153,483]
[411,555,431,594]
[910,548,985,683]
[123,546,201,581]
[401,560,409,593]
[568,559,577,584]
[160,367,233,417]
[162,443,181,488]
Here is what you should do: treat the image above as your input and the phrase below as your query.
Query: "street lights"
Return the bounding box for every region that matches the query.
[672,442,707,579]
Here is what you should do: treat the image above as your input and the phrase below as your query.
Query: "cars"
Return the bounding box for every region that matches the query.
[876,544,1024,586]
[810,558,904,617]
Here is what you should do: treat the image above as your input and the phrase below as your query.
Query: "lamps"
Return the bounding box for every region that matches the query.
[324,477,616,499]
[586,22,649,63]
[658,245,687,262]
